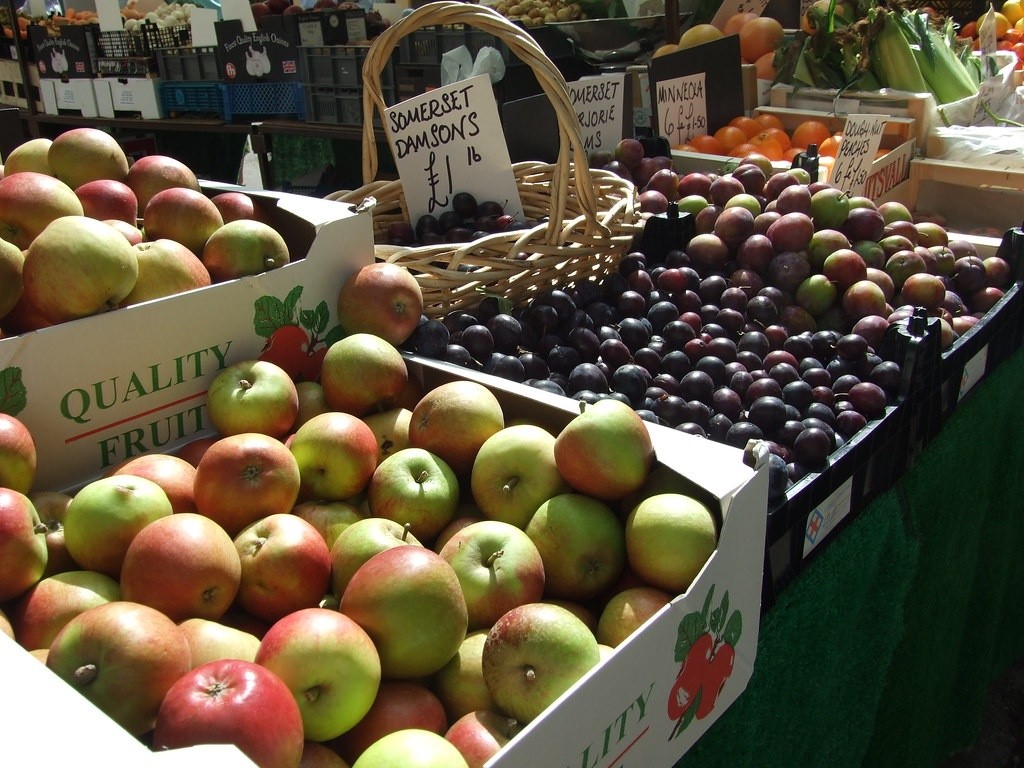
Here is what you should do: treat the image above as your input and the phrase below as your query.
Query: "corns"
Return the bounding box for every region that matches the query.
[797,6,980,111]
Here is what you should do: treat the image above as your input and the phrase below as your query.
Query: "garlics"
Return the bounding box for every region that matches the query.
[122,1,200,47]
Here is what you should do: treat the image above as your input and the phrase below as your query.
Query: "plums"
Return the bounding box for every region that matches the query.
[380,192,549,246]
[586,137,1010,356]
[412,248,905,496]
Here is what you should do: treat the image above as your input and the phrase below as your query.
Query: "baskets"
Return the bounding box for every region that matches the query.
[321,1,645,321]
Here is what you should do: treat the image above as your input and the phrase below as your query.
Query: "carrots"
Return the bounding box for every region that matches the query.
[4,0,146,39]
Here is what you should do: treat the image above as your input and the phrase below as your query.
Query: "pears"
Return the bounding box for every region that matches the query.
[975,0,1024,40]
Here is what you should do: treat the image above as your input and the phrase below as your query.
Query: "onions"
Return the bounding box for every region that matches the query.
[474,0,580,27]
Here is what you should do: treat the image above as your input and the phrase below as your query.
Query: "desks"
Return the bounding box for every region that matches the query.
[0,104,386,191]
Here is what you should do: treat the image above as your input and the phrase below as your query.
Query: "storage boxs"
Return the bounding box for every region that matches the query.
[393,57,573,124]
[0,179,376,495]
[398,19,571,69]
[39,79,98,117]
[213,15,301,81]
[283,8,368,46]
[219,81,306,122]
[59,357,770,768]
[0,59,46,114]
[401,315,932,608]
[157,81,223,120]
[90,26,192,78]
[900,225,1024,456]
[155,45,221,83]
[670,103,916,175]
[302,83,396,129]
[93,76,165,120]
[27,23,99,79]
[296,45,400,87]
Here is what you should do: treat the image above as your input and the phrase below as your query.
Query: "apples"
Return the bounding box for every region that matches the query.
[248,0,392,49]
[0,265,710,768]
[0,129,292,340]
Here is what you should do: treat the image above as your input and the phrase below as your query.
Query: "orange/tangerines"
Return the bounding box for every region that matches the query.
[956,22,1024,72]
[653,0,848,82]
[675,115,894,174]
